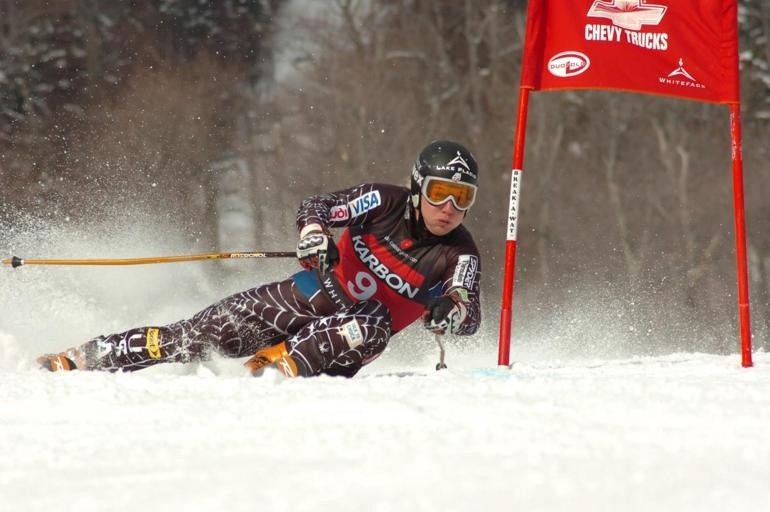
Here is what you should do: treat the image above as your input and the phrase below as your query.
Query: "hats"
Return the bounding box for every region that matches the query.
[420,175,479,211]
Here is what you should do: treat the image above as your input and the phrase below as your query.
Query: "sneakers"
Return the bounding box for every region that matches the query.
[36,349,87,373]
[244,341,298,378]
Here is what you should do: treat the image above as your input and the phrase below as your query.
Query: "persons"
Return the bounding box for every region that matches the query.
[39,139,481,379]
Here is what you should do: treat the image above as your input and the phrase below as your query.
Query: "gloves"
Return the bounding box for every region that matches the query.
[424,290,467,336]
[296,224,339,273]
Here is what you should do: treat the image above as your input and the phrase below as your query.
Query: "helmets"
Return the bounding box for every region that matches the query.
[410,140,480,194]
